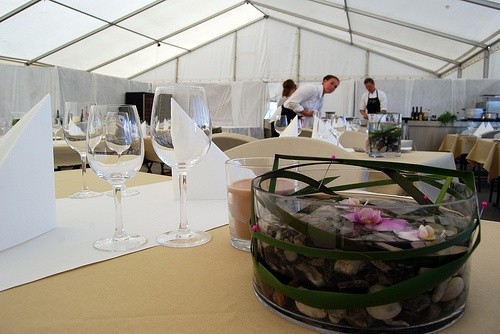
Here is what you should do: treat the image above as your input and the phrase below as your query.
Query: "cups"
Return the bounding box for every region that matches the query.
[224,157,300,252]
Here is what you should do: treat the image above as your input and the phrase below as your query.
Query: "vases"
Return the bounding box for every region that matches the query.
[253,170,476,334]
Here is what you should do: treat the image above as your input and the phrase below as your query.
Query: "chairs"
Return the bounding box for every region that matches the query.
[144,120,404,194]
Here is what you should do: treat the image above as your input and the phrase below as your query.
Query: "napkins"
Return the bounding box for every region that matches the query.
[474,121,494,135]
[311,115,344,148]
[0,93,59,251]
[279,115,299,137]
[171,96,260,200]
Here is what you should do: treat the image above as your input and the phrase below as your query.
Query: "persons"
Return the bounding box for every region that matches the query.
[282,74,340,130]
[358,77,388,120]
[268,79,304,137]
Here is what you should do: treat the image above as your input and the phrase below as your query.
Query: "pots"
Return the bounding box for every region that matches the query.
[484,113,498,119]
[464,108,484,118]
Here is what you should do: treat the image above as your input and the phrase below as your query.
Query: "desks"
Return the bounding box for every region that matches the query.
[0,133,500,334]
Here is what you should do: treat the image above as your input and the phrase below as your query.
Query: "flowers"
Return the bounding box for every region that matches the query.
[335,198,439,244]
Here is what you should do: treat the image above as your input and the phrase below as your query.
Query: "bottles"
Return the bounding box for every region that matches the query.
[411,106,436,121]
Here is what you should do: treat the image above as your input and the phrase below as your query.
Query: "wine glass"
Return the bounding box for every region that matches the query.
[466,121,474,135]
[273,114,400,157]
[0,86,212,251]
